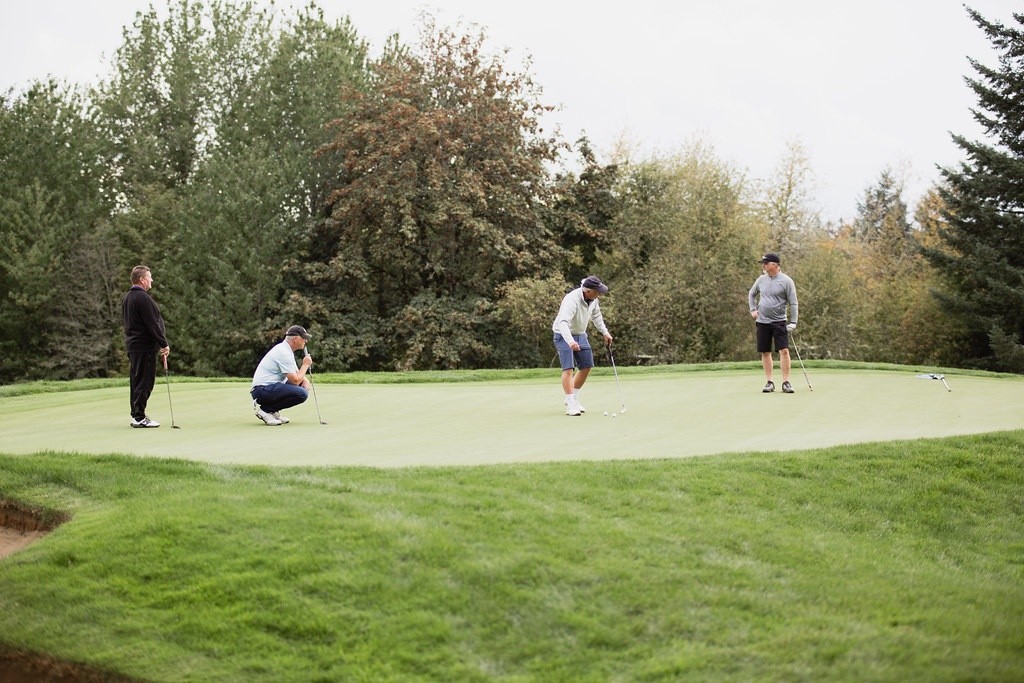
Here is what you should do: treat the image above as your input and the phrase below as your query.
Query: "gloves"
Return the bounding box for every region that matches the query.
[787,322,796,331]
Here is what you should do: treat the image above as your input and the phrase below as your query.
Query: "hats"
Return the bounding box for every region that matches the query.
[286,325,312,339]
[758,254,780,264]
[584,276,608,292]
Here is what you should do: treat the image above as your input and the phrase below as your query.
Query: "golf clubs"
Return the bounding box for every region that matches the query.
[303,346,329,425]
[606,343,628,415]
[790,331,814,392]
[162,355,181,429]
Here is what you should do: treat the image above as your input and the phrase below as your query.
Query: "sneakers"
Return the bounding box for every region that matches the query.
[565,399,584,412]
[782,381,794,393]
[763,381,774,392]
[273,411,289,423]
[130,417,159,428]
[565,401,581,416]
[255,408,282,425]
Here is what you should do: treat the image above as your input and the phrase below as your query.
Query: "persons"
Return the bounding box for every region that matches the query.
[250,325,313,426]
[748,253,798,393]
[552,276,613,415]
[122,266,170,428]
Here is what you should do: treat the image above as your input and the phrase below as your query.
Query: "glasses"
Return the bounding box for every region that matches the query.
[762,256,772,261]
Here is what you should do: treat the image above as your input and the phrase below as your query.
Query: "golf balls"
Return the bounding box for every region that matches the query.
[603,411,608,416]
[612,413,618,417]
